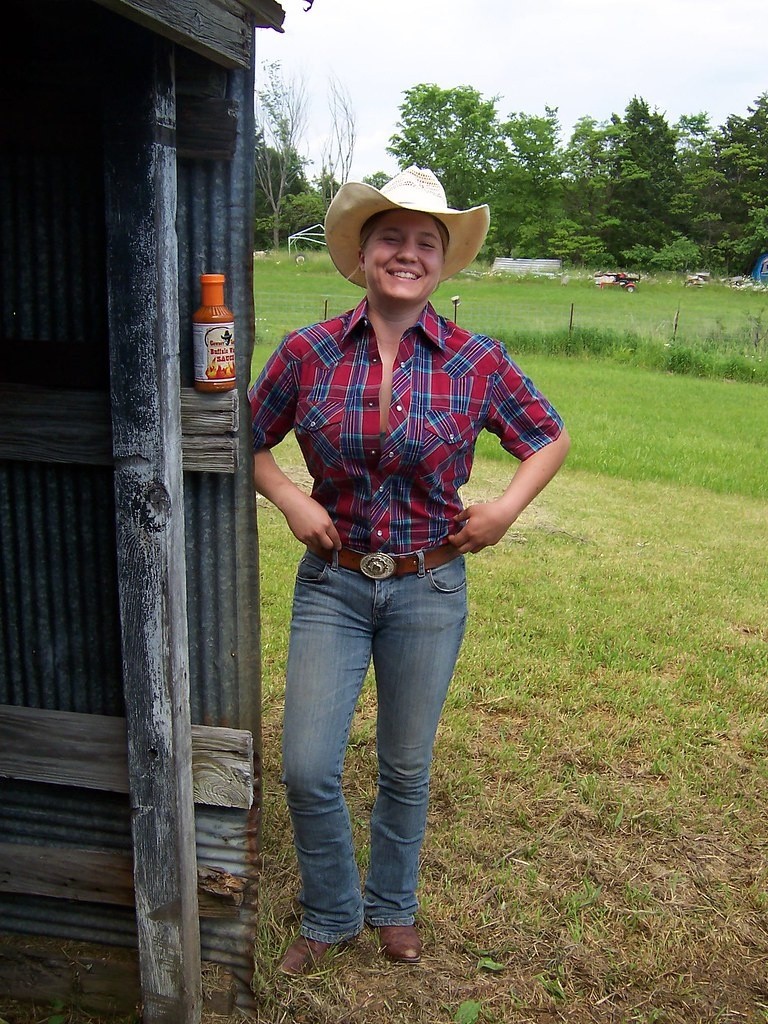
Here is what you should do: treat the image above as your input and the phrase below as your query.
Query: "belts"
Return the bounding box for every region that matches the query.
[307,542,460,581]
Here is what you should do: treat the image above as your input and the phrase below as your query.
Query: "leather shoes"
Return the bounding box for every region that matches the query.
[380,924,423,962]
[275,936,358,977]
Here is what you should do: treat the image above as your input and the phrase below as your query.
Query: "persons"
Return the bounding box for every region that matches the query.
[249,167,571,979]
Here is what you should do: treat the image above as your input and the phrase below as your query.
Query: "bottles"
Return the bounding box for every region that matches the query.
[193,275,234,392]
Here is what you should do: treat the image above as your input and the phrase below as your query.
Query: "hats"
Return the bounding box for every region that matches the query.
[325,165,490,288]
